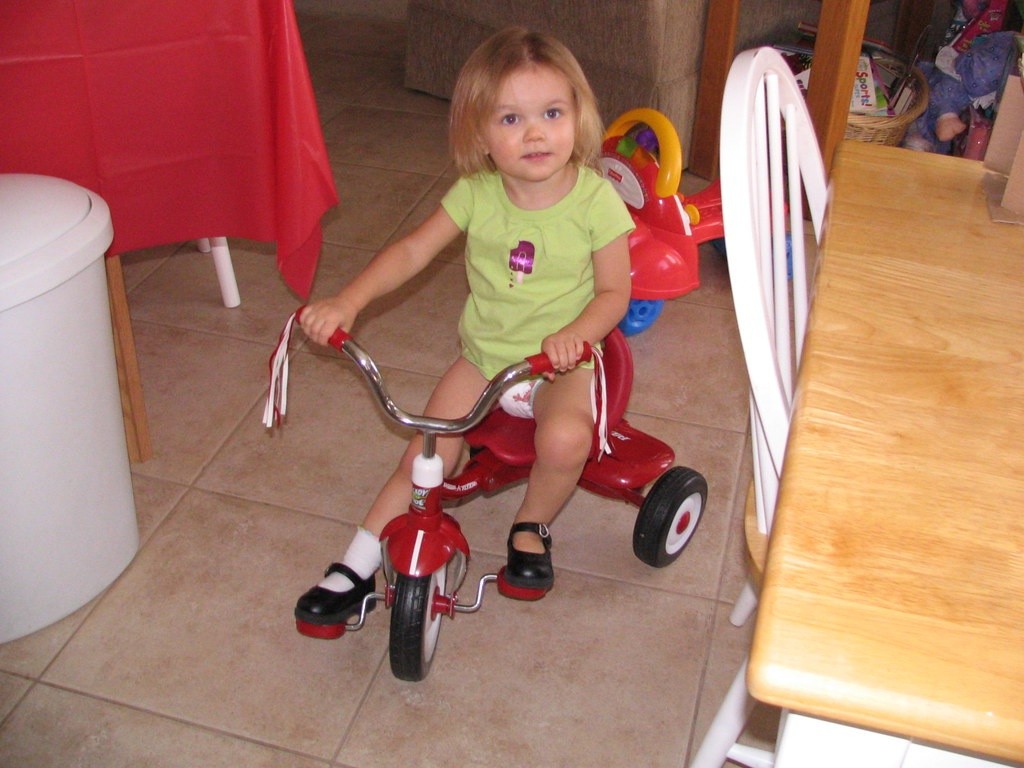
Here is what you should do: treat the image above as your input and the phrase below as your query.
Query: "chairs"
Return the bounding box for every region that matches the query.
[686,46,829,768]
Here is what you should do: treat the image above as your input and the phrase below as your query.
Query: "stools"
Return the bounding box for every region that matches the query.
[0,175,141,643]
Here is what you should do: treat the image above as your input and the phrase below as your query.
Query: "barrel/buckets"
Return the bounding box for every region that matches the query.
[0,174,139,644]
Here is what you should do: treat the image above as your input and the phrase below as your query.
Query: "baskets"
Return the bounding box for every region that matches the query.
[843,50,928,146]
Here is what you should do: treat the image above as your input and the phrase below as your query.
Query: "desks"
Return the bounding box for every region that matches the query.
[748,141,1024,768]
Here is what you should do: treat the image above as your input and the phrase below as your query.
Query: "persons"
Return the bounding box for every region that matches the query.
[291,23,637,628]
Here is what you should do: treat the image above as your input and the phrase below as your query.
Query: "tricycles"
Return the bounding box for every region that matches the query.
[293,306,708,683]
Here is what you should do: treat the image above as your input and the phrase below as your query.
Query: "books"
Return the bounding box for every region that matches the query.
[762,20,918,118]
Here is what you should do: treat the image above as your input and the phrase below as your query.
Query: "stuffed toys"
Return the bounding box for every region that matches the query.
[904,28,1024,154]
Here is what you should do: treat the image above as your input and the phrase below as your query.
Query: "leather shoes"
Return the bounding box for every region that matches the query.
[294,563,377,626]
[503,521,555,589]
